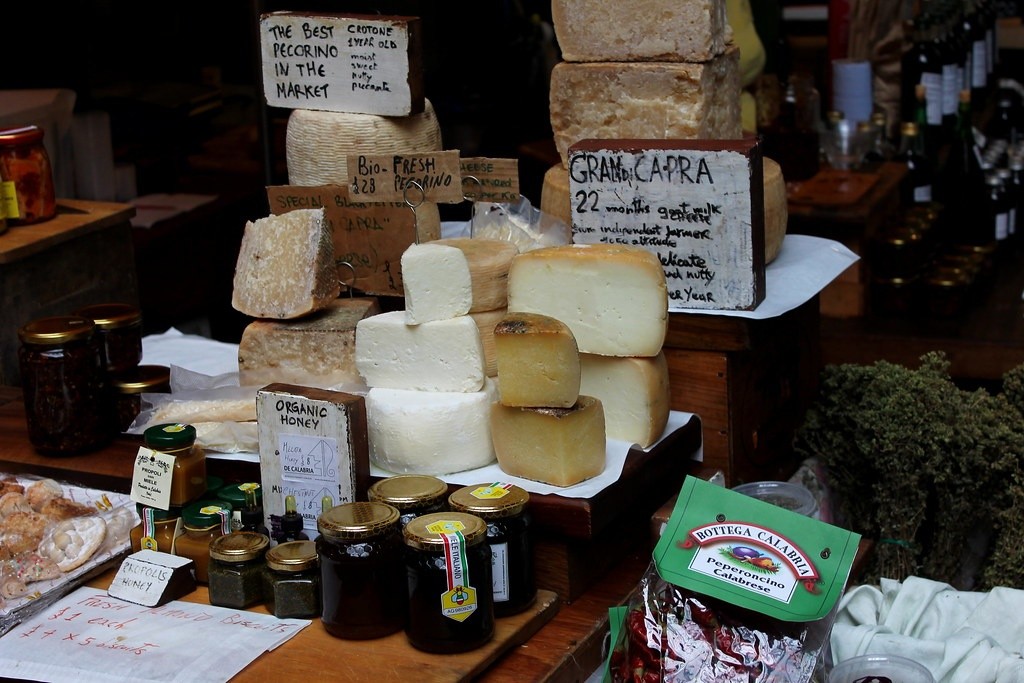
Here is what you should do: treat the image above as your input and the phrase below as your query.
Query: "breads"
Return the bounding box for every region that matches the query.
[0,475,107,611]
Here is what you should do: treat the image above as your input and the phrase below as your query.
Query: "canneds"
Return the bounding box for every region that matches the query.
[314,474,537,654]
[18,304,167,460]
[129,422,323,619]
[0,124,56,239]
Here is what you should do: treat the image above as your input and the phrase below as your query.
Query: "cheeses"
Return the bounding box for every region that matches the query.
[155,0,788,489]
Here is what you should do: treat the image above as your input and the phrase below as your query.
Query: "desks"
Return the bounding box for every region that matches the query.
[787,160,911,318]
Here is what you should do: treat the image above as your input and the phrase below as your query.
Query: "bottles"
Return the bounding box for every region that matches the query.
[822,10,1024,278]
